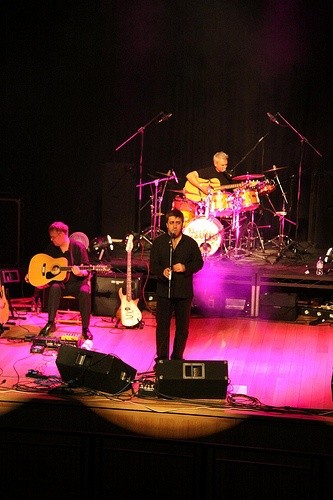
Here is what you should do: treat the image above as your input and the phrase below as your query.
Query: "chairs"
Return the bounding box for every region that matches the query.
[54,295,84,325]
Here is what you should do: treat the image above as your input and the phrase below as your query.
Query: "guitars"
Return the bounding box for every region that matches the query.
[28,253,111,289]
[184,176,261,204]
[115,233,144,327]
[0,278,9,325]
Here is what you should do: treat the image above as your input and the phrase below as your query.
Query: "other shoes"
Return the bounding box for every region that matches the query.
[153,356,168,370]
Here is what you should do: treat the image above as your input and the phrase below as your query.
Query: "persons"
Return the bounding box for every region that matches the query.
[25,221,94,340]
[186,151,232,195]
[149,209,203,371]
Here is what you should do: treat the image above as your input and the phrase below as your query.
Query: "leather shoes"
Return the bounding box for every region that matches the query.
[82,328,93,339]
[39,323,56,337]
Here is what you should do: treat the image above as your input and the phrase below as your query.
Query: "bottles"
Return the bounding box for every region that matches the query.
[315,256,324,277]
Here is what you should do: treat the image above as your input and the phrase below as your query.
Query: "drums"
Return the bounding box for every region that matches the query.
[204,191,236,219]
[230,187,261,215]
[259,179,277,197]
[174,200,201,229]
[183,214,225,258]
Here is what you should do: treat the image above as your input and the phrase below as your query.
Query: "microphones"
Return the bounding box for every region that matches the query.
[266,112,280,124]
[172,171,179,184]
[158,113,172,123]
[169,231,174,243]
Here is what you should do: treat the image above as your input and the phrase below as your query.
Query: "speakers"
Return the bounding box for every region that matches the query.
[157,360,228,399]
[0,198,24,266]
[95,277,140,317]
[56,345,137,393]
[102,163,136,237]
[307,170,333,248]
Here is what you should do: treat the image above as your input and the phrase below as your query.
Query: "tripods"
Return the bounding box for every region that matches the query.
[217,210,306,265]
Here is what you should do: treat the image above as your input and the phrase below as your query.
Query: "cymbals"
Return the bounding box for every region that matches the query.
[231,174,265,181]
[167,189,185,194]
[262,166,288,174]
